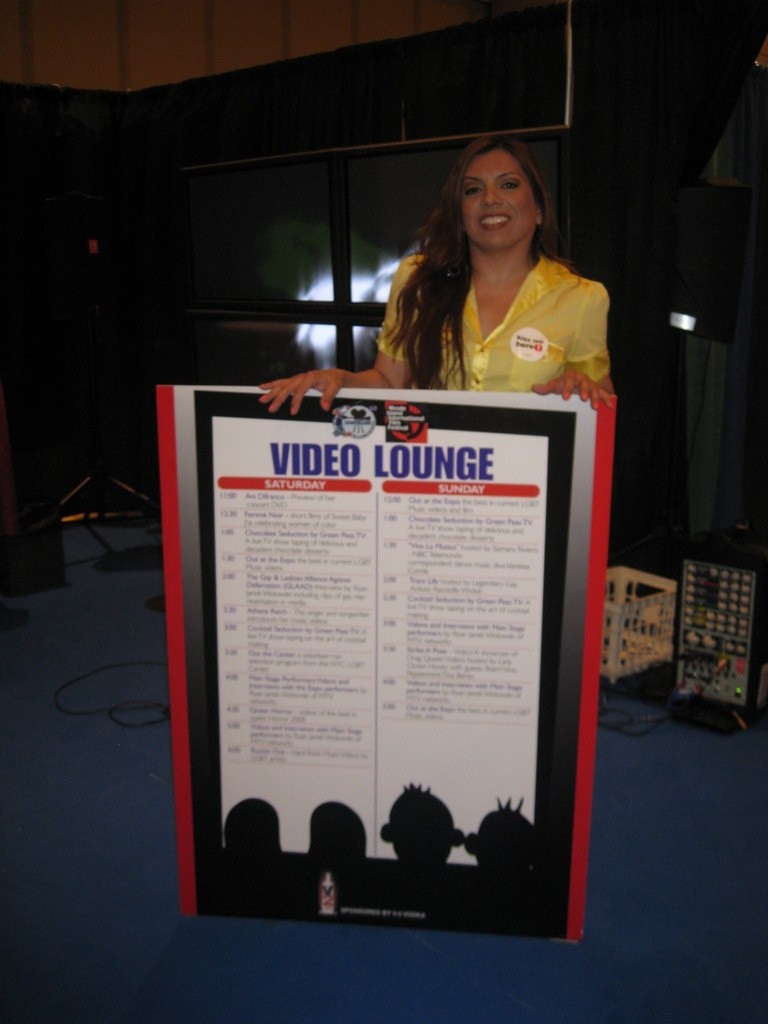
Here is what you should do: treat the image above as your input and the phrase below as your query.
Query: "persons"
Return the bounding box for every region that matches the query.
[259,135,616,415]
